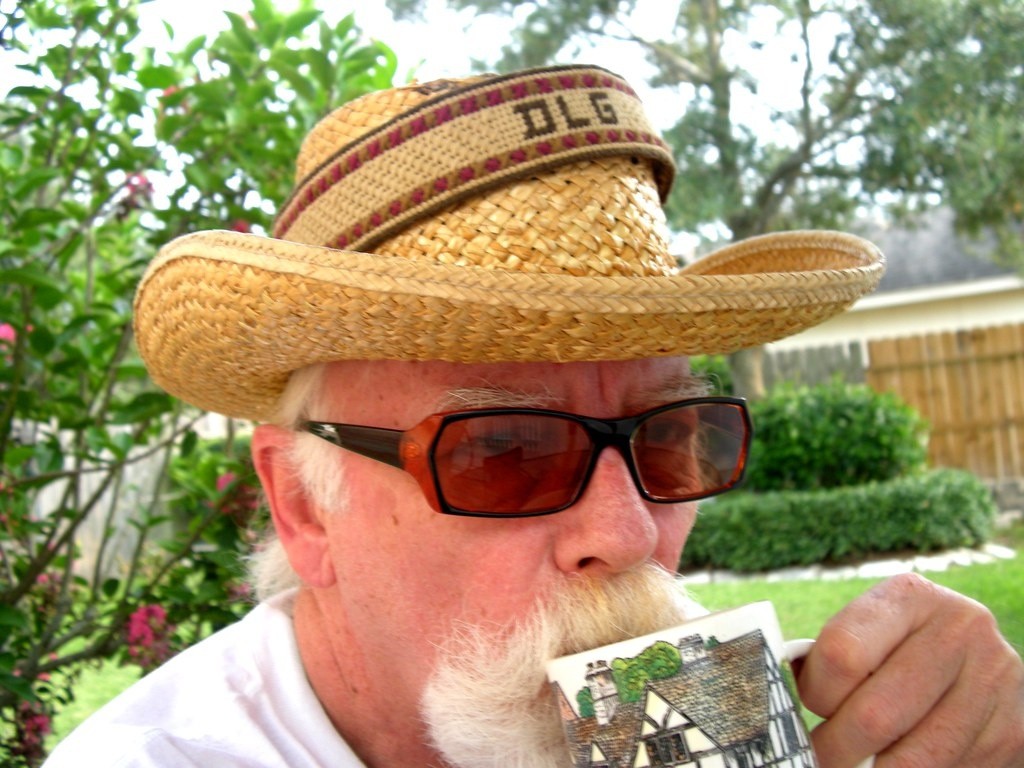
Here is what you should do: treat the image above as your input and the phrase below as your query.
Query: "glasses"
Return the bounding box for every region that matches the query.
[296,397,755,518]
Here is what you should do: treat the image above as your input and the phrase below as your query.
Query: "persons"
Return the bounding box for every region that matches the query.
[43,65,1024,768]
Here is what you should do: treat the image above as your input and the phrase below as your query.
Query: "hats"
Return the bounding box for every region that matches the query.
[132,63,887,421]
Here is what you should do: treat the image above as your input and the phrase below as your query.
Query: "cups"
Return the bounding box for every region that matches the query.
[546,600,875,768]
